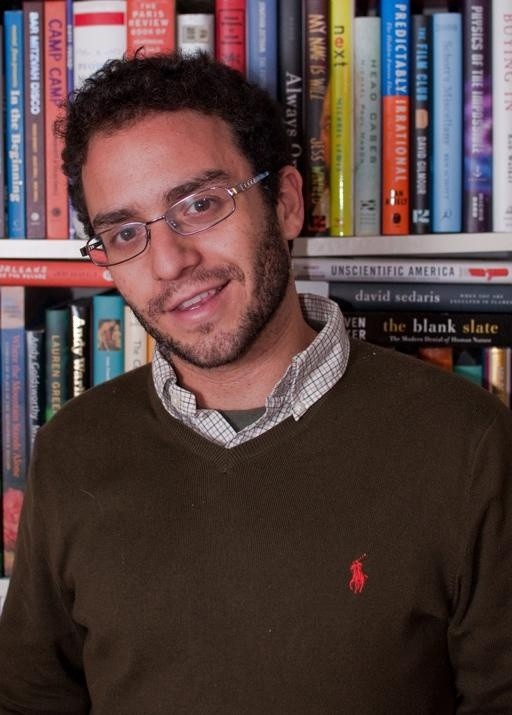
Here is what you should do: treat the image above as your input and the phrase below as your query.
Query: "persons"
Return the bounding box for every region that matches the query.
[0,44,511,705]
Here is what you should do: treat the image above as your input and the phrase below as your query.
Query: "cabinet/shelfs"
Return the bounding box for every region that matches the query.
[0,231,512,615]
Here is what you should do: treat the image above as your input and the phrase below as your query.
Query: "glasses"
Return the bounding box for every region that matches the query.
[79,171,278,267]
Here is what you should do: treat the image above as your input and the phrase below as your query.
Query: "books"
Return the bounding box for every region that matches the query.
[2,2,512,239]
[2,256,511,583]
